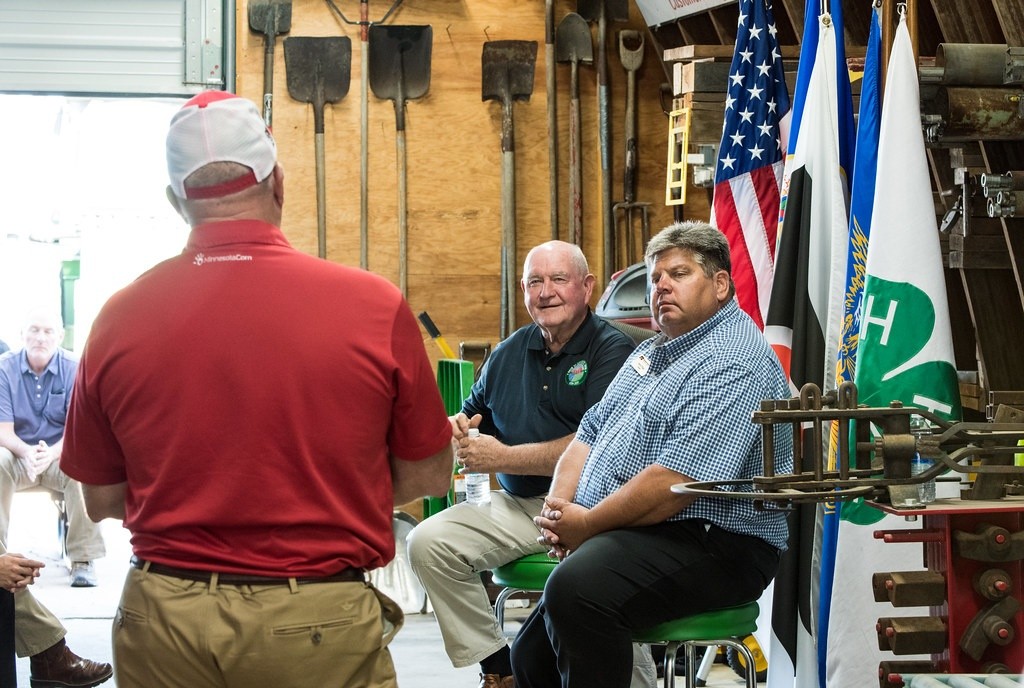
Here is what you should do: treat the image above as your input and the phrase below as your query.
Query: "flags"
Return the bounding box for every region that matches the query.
[705,0,967,687]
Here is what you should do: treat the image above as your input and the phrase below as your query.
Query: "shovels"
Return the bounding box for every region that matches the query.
[244,1,293,133]
[479,39,538,338]
[366,22,435,301]
[283,35,353,259]
[555,12,596,247]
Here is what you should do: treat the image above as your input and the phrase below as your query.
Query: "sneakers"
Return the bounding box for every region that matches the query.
[69,560,97,586]
[477,673,516,688]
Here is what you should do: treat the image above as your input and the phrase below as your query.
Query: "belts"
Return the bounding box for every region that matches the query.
[130,553,365,584]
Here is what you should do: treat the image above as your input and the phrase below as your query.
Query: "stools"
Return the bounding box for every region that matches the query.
[489,552,562,632]
[13,485,67,559]
[632,602,760,688]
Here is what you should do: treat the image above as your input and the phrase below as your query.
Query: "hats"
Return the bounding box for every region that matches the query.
[166,89,277,200]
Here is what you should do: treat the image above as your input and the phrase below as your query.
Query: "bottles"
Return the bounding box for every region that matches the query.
[909,413,936,503]
[463,428,491,505]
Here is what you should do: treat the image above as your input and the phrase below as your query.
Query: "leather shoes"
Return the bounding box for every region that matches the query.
[30,646,113,688]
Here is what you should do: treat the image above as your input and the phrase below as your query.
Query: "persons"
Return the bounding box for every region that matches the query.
[0,309,107,589]
[506,221,796,688]
[0,539,113,688]
[59,86,454,688]
[404,239,636,688]
